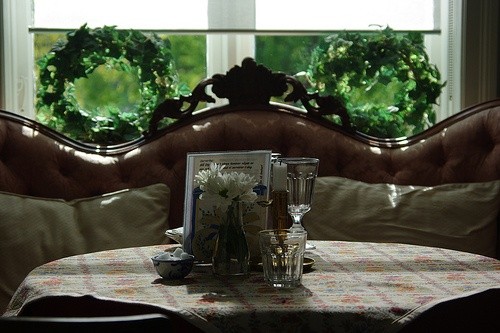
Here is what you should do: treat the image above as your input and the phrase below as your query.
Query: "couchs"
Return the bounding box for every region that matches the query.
[0,56,500,246]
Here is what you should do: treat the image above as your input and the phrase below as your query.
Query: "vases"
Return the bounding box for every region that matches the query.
[210,201,252,278]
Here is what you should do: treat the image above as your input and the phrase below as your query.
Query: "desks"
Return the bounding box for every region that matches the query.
[3,240,500,333]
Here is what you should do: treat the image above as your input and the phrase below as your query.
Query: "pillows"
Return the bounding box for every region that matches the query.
[302,178,500,256]
[0,183,172,312]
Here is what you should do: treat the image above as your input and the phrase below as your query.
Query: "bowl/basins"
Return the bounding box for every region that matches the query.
[151,253,194,280]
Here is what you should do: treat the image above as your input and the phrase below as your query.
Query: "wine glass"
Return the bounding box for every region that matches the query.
[283,157,319,250]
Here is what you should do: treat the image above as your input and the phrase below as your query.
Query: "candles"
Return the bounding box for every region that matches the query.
[271,158,288,192]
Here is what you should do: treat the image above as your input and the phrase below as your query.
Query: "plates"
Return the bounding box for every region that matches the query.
[303,257,315,271]
[258,229,307,288]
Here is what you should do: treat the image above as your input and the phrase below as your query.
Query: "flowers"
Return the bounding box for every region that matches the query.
[37,21,191,148]
[301,24,442,138]
[193,162,261,207]
